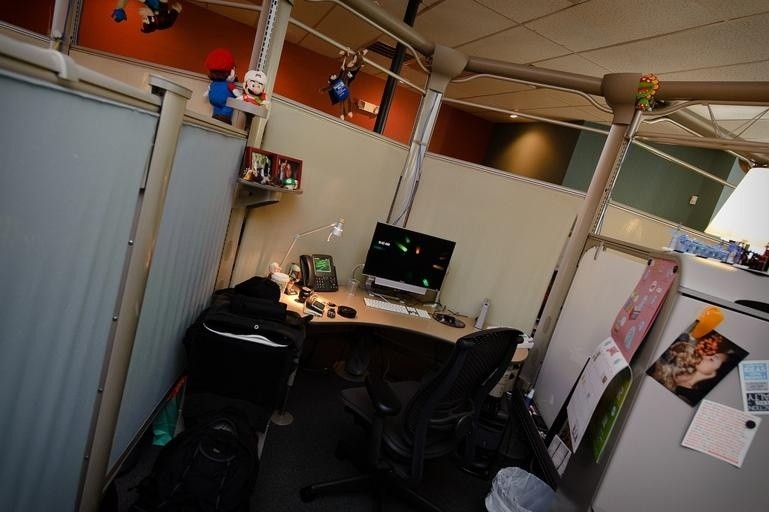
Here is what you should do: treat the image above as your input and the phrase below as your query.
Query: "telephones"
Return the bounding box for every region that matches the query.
[299,254,338,291]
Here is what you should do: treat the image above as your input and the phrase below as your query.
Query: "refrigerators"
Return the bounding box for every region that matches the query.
[540,233,767,512]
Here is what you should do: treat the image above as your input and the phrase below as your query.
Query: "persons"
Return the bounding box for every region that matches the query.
[661,352,738,406]
[253,153,296,187]
[202,48,241,125]
[238,70,272,109]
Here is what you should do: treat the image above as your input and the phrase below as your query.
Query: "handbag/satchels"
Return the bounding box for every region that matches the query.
[140,416,251,510]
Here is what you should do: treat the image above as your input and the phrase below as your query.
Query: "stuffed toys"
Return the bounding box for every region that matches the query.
[317,48,353,121]
[635,72,662,113]
[111,0,182,33]
[345,48,369,86]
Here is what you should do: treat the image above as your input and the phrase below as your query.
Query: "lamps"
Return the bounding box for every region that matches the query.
[279,215,345,268]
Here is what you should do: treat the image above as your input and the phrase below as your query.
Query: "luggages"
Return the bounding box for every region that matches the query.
[185,298,305,435]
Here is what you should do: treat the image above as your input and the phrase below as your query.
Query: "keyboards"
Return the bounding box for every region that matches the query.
[364,297,431,319]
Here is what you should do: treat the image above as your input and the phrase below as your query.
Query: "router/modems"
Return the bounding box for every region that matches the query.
[475,299,490,330]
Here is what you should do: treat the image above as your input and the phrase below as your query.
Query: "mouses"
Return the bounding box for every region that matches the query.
[448,317,455,324]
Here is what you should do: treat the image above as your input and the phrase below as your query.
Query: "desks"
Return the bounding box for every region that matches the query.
[278,284,529,363]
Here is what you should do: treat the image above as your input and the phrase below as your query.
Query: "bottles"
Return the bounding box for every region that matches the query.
[299,286,310,304]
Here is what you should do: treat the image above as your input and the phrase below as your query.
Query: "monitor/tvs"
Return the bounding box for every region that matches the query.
[361,221,456,302]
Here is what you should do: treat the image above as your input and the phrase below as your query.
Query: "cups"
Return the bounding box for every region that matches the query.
[346,278,360,297]
[271,273,290,302]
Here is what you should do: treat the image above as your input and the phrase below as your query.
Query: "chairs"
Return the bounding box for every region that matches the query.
[300,325,524,512]
